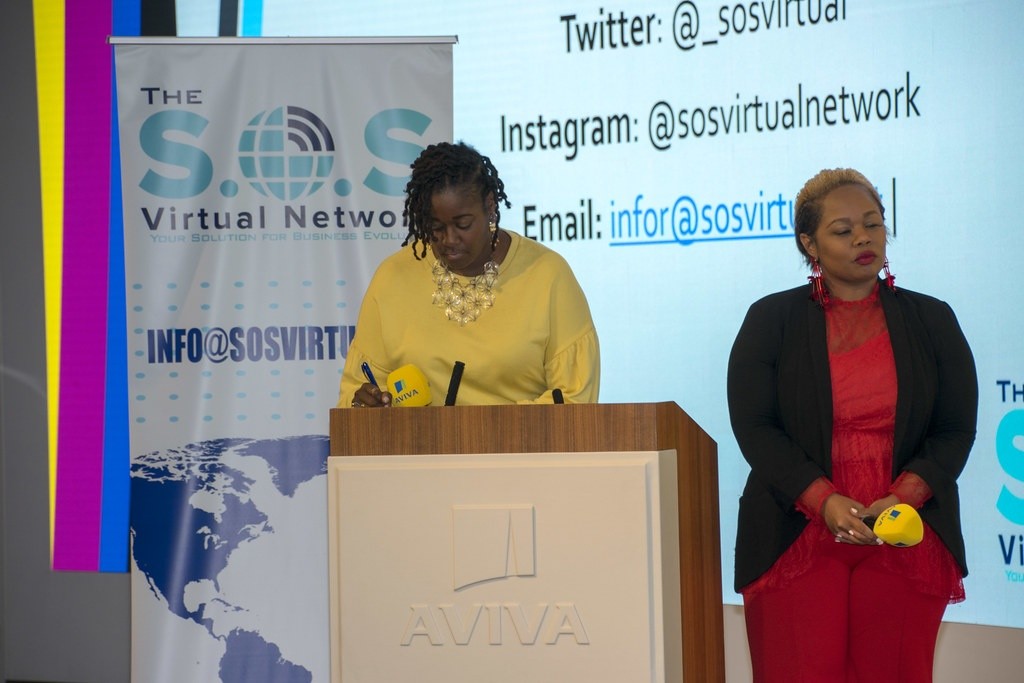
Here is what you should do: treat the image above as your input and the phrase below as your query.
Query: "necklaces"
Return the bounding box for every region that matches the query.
[430,257,499,324]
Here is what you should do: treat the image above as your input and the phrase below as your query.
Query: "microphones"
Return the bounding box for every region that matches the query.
[552,388,564,404]
[386,364,433,407]
[444,360,466,406]
[858,504,924,548]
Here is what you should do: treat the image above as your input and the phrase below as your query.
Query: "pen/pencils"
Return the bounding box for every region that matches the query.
[361,362,378,387]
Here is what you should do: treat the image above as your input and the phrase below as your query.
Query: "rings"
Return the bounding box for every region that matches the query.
[352,402,360,407]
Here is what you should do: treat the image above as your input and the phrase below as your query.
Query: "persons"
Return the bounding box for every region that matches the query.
[727,167,979,683]
[337,141,600,409]
